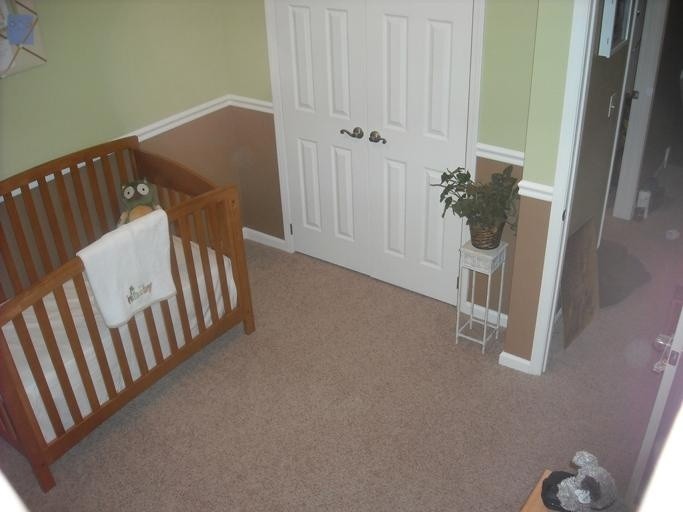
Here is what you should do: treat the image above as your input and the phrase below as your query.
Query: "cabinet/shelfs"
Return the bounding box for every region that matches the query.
[454,239,508,355]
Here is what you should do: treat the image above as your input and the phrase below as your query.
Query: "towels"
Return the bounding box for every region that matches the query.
[75,208,177,330]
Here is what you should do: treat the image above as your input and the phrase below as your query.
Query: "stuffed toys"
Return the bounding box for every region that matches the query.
[117,176,160,231]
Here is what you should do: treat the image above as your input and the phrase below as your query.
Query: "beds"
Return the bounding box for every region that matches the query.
[0,136,255,493]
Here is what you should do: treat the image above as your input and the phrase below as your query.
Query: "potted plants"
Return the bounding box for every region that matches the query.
[427,163,521,250]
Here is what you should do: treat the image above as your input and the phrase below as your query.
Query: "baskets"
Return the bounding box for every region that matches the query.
[468,219,504,250]
